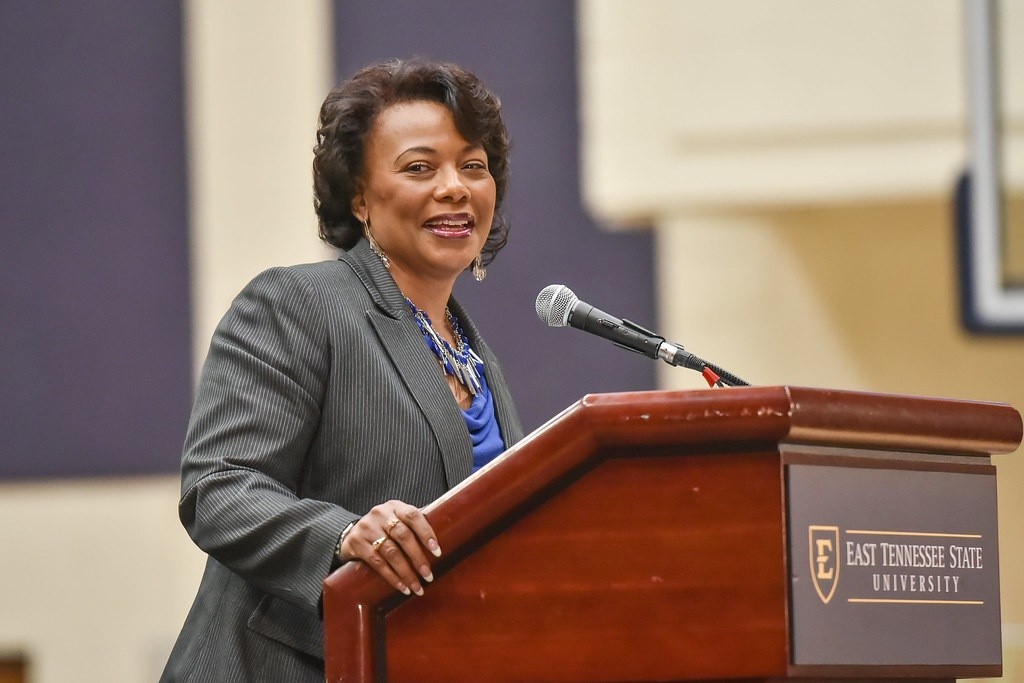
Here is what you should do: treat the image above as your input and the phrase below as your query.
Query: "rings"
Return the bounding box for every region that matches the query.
[385,518,402,535]
[372,536,391,552]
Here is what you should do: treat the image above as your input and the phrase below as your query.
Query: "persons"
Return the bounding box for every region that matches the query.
[154,53,527,683]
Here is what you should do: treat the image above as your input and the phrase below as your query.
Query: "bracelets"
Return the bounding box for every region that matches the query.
[335,520,361,563]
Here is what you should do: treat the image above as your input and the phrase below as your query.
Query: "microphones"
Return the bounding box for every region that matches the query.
[535,284,707,373]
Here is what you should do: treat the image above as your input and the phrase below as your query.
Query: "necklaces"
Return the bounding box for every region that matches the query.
[399,291,484,397]
